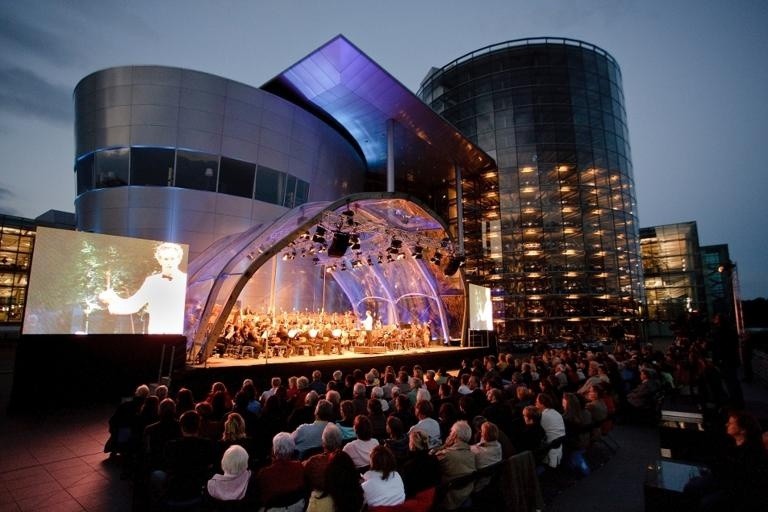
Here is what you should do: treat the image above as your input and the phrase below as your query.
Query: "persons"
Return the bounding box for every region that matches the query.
[99,242,188,334]
[108,307,768,512]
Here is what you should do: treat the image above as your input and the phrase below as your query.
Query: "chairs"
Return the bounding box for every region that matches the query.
[117,308,677,512]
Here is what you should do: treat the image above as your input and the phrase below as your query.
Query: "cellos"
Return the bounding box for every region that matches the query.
[341,327,349,346]
[356,330,367,345]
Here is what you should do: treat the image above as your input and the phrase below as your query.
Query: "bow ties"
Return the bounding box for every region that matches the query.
[162,274,173,281]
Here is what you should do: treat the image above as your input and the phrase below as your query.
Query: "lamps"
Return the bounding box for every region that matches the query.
[282,201,447,273]
